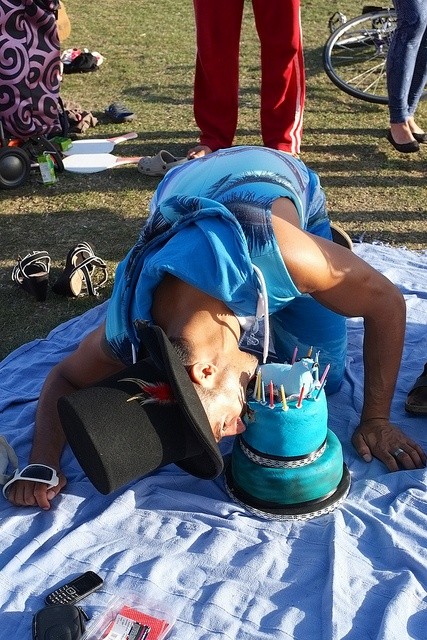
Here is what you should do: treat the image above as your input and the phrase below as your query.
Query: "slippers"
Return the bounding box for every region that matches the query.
[137,150,189,176]
[104,103,137,124]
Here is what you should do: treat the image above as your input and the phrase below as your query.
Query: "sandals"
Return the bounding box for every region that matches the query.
[10,251,53,302]
[58,239,108,301]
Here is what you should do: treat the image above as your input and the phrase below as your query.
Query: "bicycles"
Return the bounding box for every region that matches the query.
[322,5,397,105]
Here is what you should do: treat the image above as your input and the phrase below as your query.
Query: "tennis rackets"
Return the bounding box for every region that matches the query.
[61,132,138,156]
[62,153,144,175]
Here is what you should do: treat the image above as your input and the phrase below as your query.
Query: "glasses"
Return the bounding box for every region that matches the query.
[2,464,60,500]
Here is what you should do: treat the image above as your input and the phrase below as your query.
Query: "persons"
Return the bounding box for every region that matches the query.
[9,145,427,511]
[384,0,427,153]
[188,0,306,160]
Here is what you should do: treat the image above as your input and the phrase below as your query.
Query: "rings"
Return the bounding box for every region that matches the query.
[395,448,403,454]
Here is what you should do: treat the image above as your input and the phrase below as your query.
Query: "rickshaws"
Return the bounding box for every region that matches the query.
[0,0,70,188]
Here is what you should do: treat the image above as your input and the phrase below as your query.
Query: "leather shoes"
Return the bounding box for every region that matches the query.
[383,127,420,155]
[413,130,427,144]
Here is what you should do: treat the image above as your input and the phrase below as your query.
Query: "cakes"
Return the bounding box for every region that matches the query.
[224,357,352,522]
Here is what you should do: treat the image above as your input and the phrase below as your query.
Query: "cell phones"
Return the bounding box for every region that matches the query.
[44,571,104,606]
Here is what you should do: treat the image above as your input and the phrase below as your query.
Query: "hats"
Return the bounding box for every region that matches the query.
[56,322,226,495]
